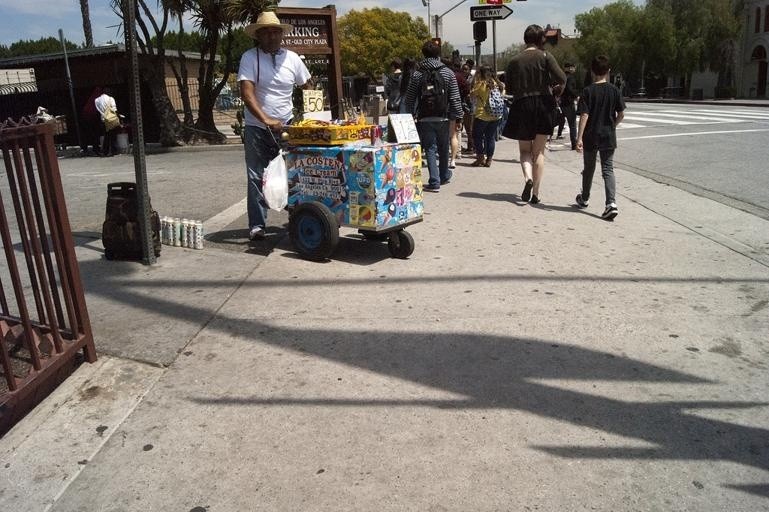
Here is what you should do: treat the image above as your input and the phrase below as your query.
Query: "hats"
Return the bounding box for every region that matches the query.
[245,11,293,39]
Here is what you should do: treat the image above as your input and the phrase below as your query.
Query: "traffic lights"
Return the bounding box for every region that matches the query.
[474,22,487,42]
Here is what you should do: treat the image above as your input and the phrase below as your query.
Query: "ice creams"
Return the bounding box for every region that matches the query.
[383,203,397,226]
[382,167,394,189]
[382,149,392,169]
[330,189,349,208]
[382,188,396,206]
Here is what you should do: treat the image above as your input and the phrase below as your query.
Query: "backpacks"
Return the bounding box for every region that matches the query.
[480,84,505,120]
[416,65,448,119]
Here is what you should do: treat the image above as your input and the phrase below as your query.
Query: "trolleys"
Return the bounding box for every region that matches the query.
[267,117,422,261]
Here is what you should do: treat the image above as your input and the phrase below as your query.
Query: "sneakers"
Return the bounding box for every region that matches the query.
[576,194,589,209]
[250,225,264,240]
[423,184,439,191]
[450,161,455,168]
[602,204,618,219]
[448,161,450,167]
[441,178,449,184]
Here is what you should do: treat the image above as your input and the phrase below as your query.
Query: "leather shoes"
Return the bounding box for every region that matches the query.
[486,157,492,166]
[531,195,540,204]
[521,179,534,201]
[472,156,485,165]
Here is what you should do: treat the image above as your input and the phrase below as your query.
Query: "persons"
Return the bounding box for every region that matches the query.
[457,127,462,159]
[453,57,461,70]
[466,59,474,71]
[500,73,509,140]
[440,57,469,168]
[470,64,505,167]
[502,24,567,204]
[615,76,621,89]
[404,41,463,192]
[575,55,626,219]
[399,56,417,114]
[557,64,580,149]
[81,86,103,156]
[237,12,311,242]
[383,57,403,112]
[94,87,120,155]
[462,64,474,154]
[568,64,576,74]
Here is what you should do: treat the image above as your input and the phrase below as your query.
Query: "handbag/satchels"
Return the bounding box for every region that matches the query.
[103,97,120,131]
[542,51,558,110]
